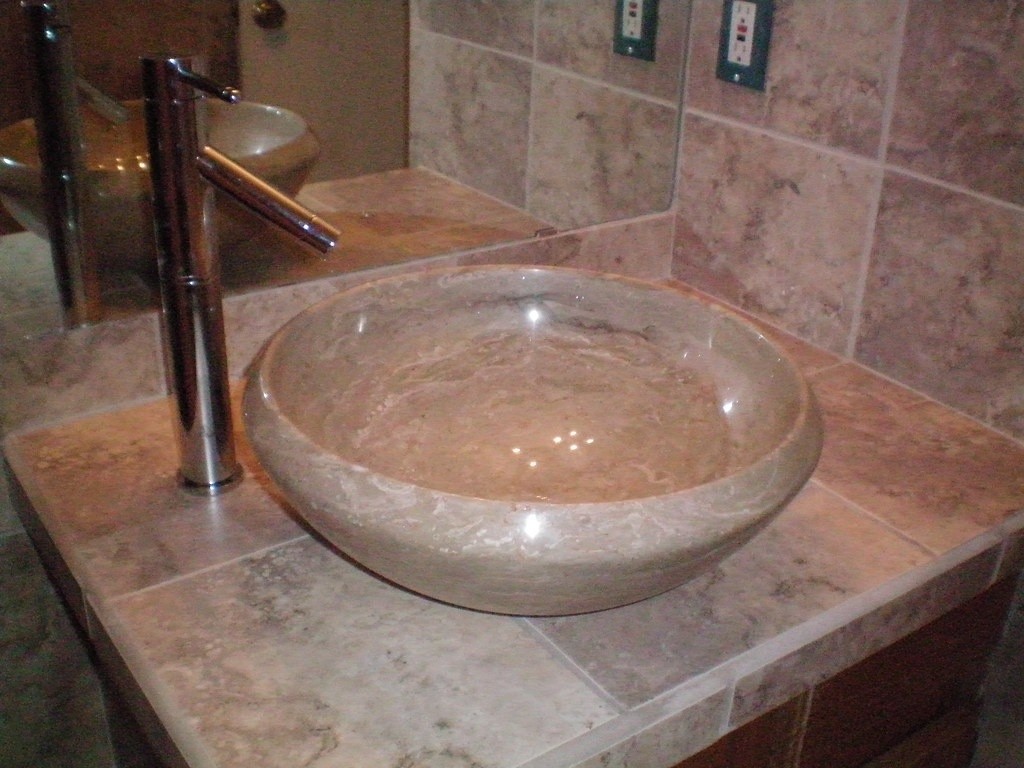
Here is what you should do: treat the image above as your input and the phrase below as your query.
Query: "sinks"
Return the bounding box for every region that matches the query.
[0,96,320,266]
[242,265,827,618]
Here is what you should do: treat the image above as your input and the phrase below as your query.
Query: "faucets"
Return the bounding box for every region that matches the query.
[137,43,344,491]
[17,1,130,329]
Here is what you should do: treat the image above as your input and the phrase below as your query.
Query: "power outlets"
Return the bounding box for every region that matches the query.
[715,0,775,93]
[613,0,660,63]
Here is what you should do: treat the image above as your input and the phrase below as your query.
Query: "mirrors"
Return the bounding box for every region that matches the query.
[55,0,690,326]
